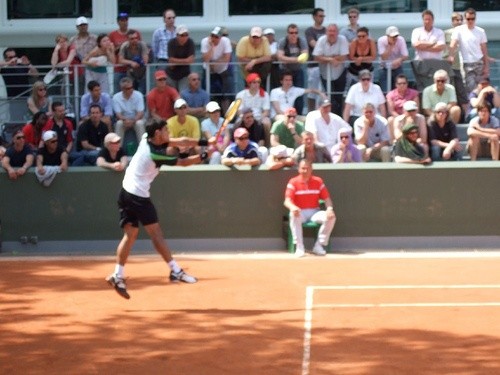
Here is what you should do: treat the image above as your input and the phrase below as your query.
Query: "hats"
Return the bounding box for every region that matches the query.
[42,131,57,142]
[76,16,87,25]
[359,69,371,80]
[174,98,187,107]
[404,102,418,110]
[273,144,294,157]
[211,27,222,37]
[286,107,296,114]
[206,101,220,111]
[319,96,331,106]
[239,107,251,120]
[234,128,249,137]
[103,133,121,148]
[155,70,167,79]
[386,26,398,37]
[480,77,490,84]
[264,29,274,34]
[246,73,259,83]
[179,27,189,34]
[251,28,262,37]
[118,14,127,18]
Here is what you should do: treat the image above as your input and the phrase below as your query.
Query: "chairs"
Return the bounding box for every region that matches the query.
[285,198,334,255]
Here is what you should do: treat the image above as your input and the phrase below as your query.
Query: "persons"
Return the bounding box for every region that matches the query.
[222,108,265,151]
[51,34,85,96]
[377,26,409,92]
[312,23,349,111]
[39,101,84,166]
[202,101,228,165]
[354,104,391,162]
[24,81,53,122]
[235,27,272,90]
[428,102,463,161]
[1,129,34,179]
[344,7,366,42]
[269,72,327,125]
[0,48,39,123]
[465,76,499,123]
[467,103,500,160]
[444,13,465,105]
[269,108,305,148]
[294,132,333,164]
[82,33,116,94]
[76,105,110,165]
[388,75,420,119]
[108,13,142,93]
[266,145,296,170]
[276,24,309,114]
[22,112,47,149]
[394,101,427,142]
[68,16,99,61]
[166,99,205,164]
[392,123,432,163]
[410,10,447,89]
[112,77,146,149]
[305,7,330,50]
[236,73,272,147]
[350,27,376,75]
[118,29,148,94]
[221,127,264,166]
[36,130,68,174]
[106,119,218,299]
[152,9,178,88]
[262,28,277,62]
[305,99,353,156]
[422,69,461,124]
[331,128,363,163]
[96,132,128,171]
[165,24,196,91]
[180,72,210,120]
[343,69,386,128]
[444,8,488,94]
[283,159,336,258]
[147,71,180,124]
[200,26,232,101]
[78,81,112,131]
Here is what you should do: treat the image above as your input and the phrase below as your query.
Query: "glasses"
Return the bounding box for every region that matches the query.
[17,135,25,139]
[51,139,58,143]
[466,17,475,20]
[239,136,249,140]
[341,135,348,139]
[130,36,138,39]
[168,17,174,20]
[397,82,406,85]
[436,80,444,83]
[357,34,367,37]
[6,55,16,60]
[287,115,295,118]
[38,88,45,91]
[288,31,298,33]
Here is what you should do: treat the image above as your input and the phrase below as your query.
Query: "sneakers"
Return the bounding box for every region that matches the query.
[312,247,326,255]
[170,270,196,283]
[106,274,130,299]
[296,247,305,257]
